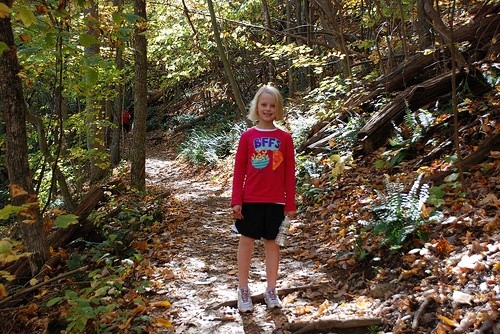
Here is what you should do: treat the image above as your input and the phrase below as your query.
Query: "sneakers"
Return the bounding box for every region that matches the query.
[237,286,254,313]
[263,290,283,309]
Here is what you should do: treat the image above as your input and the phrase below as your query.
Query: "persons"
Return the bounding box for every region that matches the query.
[123,109,131,134]
[230,86,297,314]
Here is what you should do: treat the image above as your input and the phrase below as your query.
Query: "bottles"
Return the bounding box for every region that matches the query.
[275,215,291,247]
